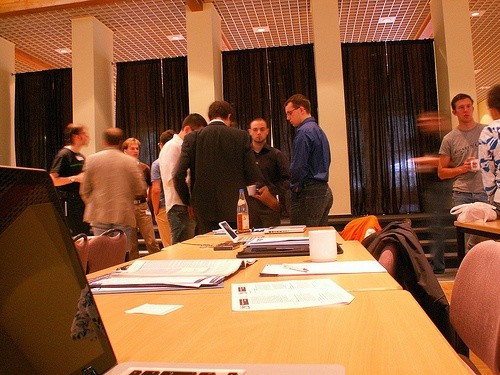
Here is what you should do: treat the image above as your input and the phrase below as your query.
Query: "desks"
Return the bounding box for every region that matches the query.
[84,223,479,375]
[454,214,500,268]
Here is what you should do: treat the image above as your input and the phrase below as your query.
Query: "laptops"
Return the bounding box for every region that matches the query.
[0,165,344,375]
[264,224,307,233]
[218,221,264,242]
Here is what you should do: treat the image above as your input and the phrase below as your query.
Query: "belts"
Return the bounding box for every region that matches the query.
[133,199,146,204]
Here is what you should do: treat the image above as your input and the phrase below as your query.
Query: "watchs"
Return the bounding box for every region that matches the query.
[69,176,75,182]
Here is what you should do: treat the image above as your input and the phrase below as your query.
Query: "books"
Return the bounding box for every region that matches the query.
[264,223,307,233]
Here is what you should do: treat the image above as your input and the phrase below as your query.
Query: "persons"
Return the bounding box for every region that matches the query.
[121,137,160,260]
[171,101,258,237]
[79,127,146,262]
[464,85,500,252]
[284,94,334,227]
[151,130,176,247]
[438,93,489,218]
[159,112,208,246]
[50,124,94,236]
[243,119,289,228]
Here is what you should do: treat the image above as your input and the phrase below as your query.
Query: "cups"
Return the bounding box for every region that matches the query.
[471,159,479,170]
[247,185,256,196]
[309,230,337,262]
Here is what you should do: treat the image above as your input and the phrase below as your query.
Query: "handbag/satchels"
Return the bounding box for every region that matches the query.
[449,199,498,226]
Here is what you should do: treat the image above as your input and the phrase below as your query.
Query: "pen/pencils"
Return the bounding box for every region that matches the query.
[284,265,307,271]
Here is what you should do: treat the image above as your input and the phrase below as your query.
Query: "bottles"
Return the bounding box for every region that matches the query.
[237,189,249,232]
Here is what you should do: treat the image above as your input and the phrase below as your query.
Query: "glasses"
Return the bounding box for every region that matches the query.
[286,106,303,116]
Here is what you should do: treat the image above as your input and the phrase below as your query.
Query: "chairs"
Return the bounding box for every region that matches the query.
[86,228,128,274]
[371,229,400,275]
[344,214,382,247]
[71,233,88,275]
[448,236,500,375]
